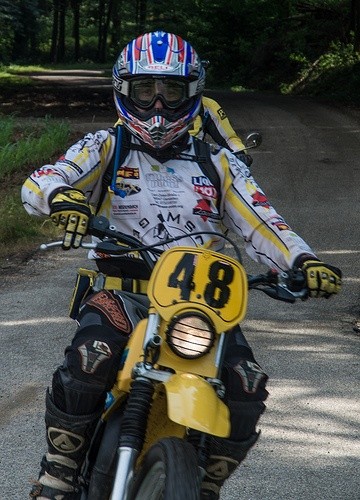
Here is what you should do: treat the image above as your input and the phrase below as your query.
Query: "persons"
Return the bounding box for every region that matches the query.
[22,32,343,500]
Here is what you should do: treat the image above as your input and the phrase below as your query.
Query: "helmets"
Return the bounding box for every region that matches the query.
[112,31,205,153]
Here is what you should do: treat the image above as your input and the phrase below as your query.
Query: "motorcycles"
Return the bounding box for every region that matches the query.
[29,214,343,499]
[230,132,263,167]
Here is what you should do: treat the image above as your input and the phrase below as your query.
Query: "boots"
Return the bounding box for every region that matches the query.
[200,429,261,500]
[29,386,104,500]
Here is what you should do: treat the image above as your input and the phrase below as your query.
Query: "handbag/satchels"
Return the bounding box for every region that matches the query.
[68,268,98,320]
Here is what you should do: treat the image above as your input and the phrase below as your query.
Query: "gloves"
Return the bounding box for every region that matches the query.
[49,188,96,251]
[236,154,253,167]
[297,257,342,302]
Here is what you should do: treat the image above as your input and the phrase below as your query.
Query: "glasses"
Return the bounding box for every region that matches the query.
[112,67,206,111]
